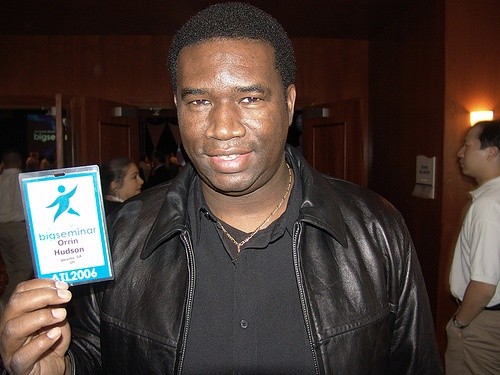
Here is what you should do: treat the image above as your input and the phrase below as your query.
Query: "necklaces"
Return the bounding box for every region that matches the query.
[216,163,292,253]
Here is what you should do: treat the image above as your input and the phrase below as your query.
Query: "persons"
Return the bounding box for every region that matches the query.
[22,149,69,172]
[145,148,168,190]
[99,148,180,218]
[0,150,33,311]
[445,120,500,375]
[0,1,447,375]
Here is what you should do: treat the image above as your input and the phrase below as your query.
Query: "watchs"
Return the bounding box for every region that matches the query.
[453,314,469,329]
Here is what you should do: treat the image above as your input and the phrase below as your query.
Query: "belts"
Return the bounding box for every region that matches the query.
[457,298,500,310]
